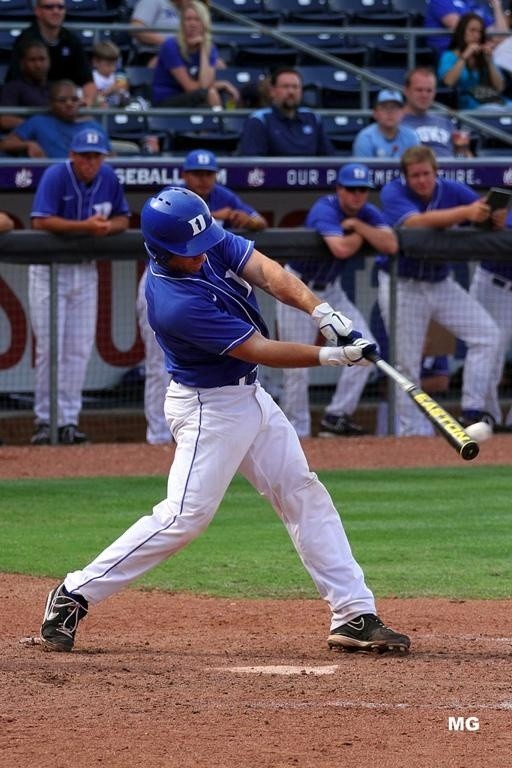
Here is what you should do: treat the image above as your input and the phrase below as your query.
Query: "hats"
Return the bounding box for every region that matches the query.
[183,149,218,173]
[338,164,374,189]
[70,128,108,155]
[377,88,405,104]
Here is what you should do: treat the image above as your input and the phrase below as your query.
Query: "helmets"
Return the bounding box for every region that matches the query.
[140,186,225,257]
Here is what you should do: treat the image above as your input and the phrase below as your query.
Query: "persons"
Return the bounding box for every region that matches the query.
[39,182,414,655]
[1,0,512,447]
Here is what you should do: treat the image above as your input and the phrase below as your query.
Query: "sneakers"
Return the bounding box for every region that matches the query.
[31,425,50,443]
[58,426,88,443]
[327,614,411,653]
[40,583,88,651]
[319,414,364,437]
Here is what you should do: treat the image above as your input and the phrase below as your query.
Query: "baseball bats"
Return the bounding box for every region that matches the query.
[367,351,479,461]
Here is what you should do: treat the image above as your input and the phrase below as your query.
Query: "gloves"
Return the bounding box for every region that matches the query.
[318,337,376,367]
[311,303,354,346]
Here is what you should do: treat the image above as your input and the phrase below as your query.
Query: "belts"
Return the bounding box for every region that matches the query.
[227,369,257,386]
[492,278,511,290]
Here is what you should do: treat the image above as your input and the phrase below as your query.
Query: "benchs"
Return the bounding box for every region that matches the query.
[0,0,512,189]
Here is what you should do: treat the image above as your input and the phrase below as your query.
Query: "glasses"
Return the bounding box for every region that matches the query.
[40,5,63,9]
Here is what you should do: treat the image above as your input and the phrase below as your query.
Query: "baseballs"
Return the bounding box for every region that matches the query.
[467,421,493,451]
